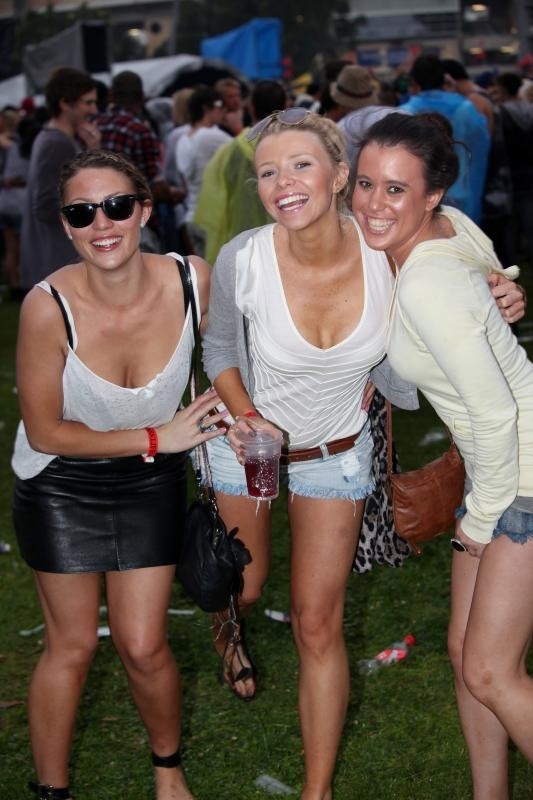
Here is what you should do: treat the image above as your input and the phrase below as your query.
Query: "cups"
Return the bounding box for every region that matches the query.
[238,429,284,501]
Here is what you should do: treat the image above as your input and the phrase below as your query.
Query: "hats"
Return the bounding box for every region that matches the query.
[110,71,142,105]
[331,65,381,107]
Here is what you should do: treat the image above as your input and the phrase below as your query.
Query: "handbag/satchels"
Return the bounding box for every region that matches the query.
[169,500,253,610]
[388,446,466,542]
[484,154,513,226]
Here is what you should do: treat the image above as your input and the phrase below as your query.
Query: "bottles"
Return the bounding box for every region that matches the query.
[355,632,415,676]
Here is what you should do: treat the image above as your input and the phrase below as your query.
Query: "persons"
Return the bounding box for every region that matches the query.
[0,52,533,800]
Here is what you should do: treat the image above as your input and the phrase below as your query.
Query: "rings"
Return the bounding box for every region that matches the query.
[451,538,466,553]
[227,428,236,433]
[235,415,239,420]
[197,420,207,432]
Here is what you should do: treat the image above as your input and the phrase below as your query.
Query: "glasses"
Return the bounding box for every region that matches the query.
[246,108,312,151]
[61,194,145,228]
[213,100,225,109]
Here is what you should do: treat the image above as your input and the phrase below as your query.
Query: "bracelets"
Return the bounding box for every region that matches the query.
[244,412,260,417]
[140,426,158,463]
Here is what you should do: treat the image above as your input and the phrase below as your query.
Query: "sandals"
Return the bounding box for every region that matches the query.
[27,782,74,800]
[152,750,194,800]
[214,622,257,701]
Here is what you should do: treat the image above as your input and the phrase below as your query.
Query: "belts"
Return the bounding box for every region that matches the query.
[209,410,370,462]
